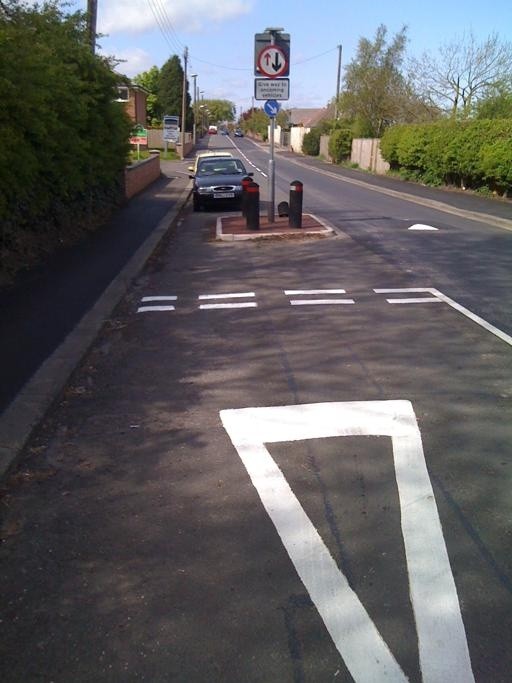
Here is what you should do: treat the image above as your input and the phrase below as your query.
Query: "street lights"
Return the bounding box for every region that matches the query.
[200,90,205,137]
[190,73,198,144]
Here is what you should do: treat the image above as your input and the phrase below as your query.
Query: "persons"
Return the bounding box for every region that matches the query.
[203,167,215,176]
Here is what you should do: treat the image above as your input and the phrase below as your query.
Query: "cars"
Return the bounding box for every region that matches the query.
[188,156,254,211]
[188,149,237,189]
[208,119,244,137]
